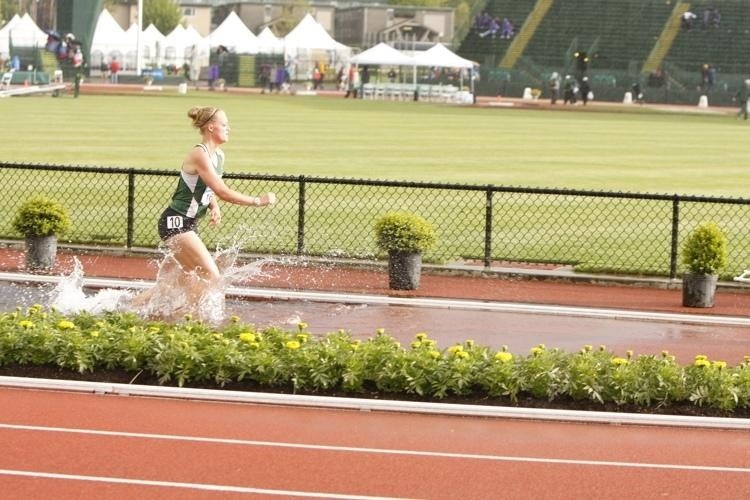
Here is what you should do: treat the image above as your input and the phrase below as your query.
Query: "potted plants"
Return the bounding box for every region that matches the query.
[675,223,723,307]
[10,195,76,274]
[374,211,434,289]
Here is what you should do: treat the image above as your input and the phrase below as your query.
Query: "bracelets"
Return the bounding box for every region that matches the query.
[254,197,261,206]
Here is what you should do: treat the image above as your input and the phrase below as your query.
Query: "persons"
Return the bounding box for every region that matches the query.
[312,68,321,89]
[548,71,559,105]
[699,63,714,92]
[474,10,515,40]
[335,66,346,90]
[119,105,279,328]
[580,76,591,107]
[276,63,285,93]
[344,63,360,99]
[575,51,587,75]
[563,74,578,105]
[677,6,722,30]
[388,68,397,83]
[99,56,109,83]
[731,78,750,121]
[258,66,270,94]
[360,66,369,83]
[647,69,663,87]
[426,63,481,82]
[107,55,120,85]
[269,63,278,93]
